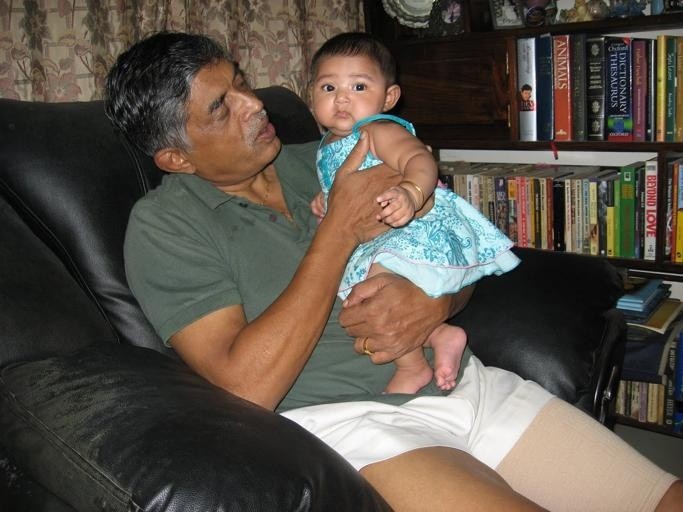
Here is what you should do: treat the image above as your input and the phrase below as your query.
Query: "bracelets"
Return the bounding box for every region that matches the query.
[393,179,426,207]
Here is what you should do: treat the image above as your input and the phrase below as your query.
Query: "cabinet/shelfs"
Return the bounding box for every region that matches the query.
[360,0,683,440]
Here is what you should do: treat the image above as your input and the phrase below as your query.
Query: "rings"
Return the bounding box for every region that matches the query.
[363,337,373,357]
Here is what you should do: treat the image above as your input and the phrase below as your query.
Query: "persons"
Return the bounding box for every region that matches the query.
[306,31,522,394]
[104,31,682,512]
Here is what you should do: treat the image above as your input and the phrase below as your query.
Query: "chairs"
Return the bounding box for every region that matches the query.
[0,85,631,512]
